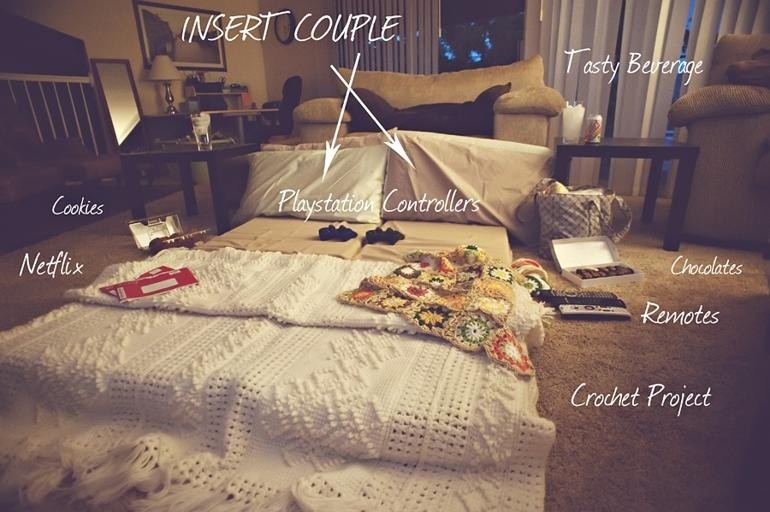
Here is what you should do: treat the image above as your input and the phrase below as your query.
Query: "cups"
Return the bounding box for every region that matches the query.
[561,100,586,147]
[197,145,213,152]
[586,116,603,144]
[191,113,211,145]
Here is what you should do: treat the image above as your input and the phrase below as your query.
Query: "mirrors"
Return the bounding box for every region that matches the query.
[89,56,154,154]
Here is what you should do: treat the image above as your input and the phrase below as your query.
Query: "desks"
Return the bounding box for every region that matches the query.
[555,136,699,256]
[199,106,281,148]
[152,137,234,150]
[124,139,260,238]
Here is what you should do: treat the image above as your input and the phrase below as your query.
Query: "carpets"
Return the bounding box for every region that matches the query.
[2,179,200,257]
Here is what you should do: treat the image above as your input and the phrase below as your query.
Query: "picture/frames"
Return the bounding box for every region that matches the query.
[131,1,229,71]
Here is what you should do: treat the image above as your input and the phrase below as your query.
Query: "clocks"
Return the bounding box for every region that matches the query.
[274,8,296,45]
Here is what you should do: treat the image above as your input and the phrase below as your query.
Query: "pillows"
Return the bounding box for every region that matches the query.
[258,127,398,218]
[381,126,553,232]
[229,145,388,239]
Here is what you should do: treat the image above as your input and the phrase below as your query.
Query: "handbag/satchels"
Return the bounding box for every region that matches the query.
[514,176,632,259]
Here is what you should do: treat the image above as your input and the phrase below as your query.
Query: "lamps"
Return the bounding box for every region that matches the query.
[142,54,183,115]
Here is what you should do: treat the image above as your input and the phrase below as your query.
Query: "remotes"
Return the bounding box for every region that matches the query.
[551,299,626,308]
[530,289,617,300]
[559,304,631,319]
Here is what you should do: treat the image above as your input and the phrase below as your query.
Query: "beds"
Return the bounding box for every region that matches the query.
[0,214,521,473]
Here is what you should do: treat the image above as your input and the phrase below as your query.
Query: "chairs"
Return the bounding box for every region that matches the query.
[668,31,770,249]
[288,52,564,144]
[265,74,303,132]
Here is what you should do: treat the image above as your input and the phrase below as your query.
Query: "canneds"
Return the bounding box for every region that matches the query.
[584,114,602,144]
